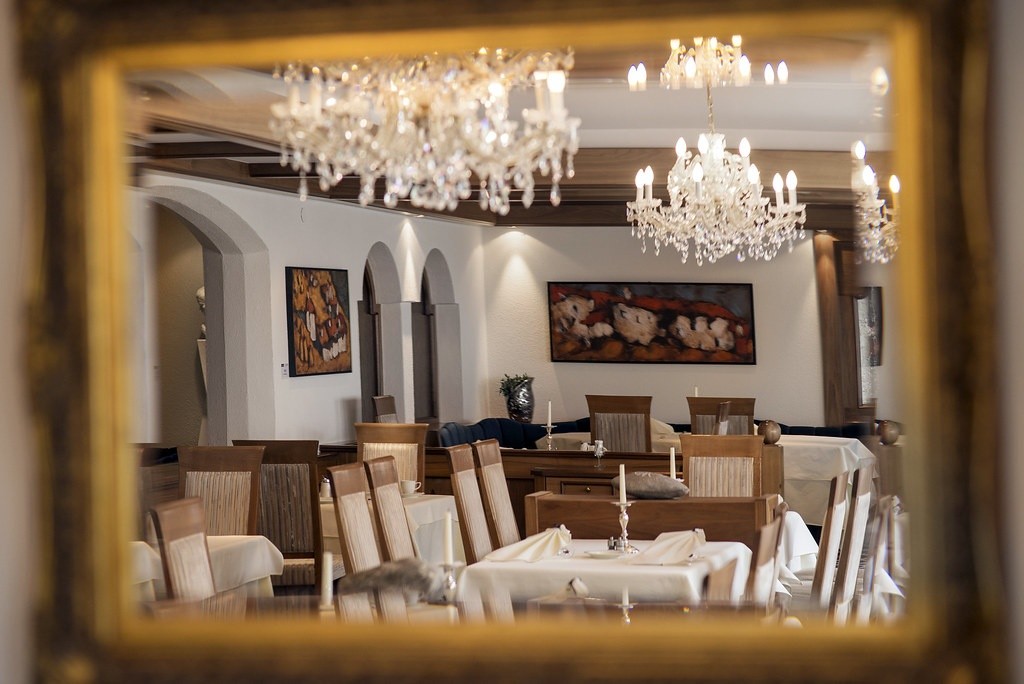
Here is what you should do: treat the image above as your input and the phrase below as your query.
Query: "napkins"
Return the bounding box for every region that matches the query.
[485,521,570,562]
[632,527,709,565]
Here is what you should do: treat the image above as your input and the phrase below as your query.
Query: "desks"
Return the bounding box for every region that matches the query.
[861,432,910,499]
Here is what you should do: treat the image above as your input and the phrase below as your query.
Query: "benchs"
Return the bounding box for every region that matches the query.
[436,410,872,449]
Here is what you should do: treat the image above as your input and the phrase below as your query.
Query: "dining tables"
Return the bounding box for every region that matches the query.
[536,432,685,453]
[778,435,878,525]
[457,538,752,616]
[206,536,283,598]
[318,495,466,566]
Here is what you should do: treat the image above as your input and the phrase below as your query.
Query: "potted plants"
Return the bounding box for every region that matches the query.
[500,373,534,422]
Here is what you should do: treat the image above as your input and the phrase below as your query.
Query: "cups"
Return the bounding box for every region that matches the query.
[401,481,421,494]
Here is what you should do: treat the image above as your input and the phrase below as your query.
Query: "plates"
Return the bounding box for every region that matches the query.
[585,550,625,560]
[400,492,426,498]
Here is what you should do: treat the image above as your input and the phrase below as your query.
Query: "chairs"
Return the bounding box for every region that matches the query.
[125,394,908,627]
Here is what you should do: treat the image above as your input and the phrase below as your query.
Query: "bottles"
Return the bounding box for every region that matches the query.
[607,536,623,551]
[321,479,331,497]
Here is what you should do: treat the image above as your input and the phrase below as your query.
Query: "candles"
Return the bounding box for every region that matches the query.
[695,386,698,397]
[548,399,552,426]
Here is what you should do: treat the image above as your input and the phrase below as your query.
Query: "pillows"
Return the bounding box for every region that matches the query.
[609,472,691,497]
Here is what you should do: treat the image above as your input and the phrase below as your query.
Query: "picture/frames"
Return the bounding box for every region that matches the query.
[285,267,352,378]
[547,280,757,365]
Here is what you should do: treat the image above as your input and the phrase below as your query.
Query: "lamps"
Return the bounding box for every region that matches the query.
[626,35,808,265]
[264,49,579,212]
[851,37,901,262]
